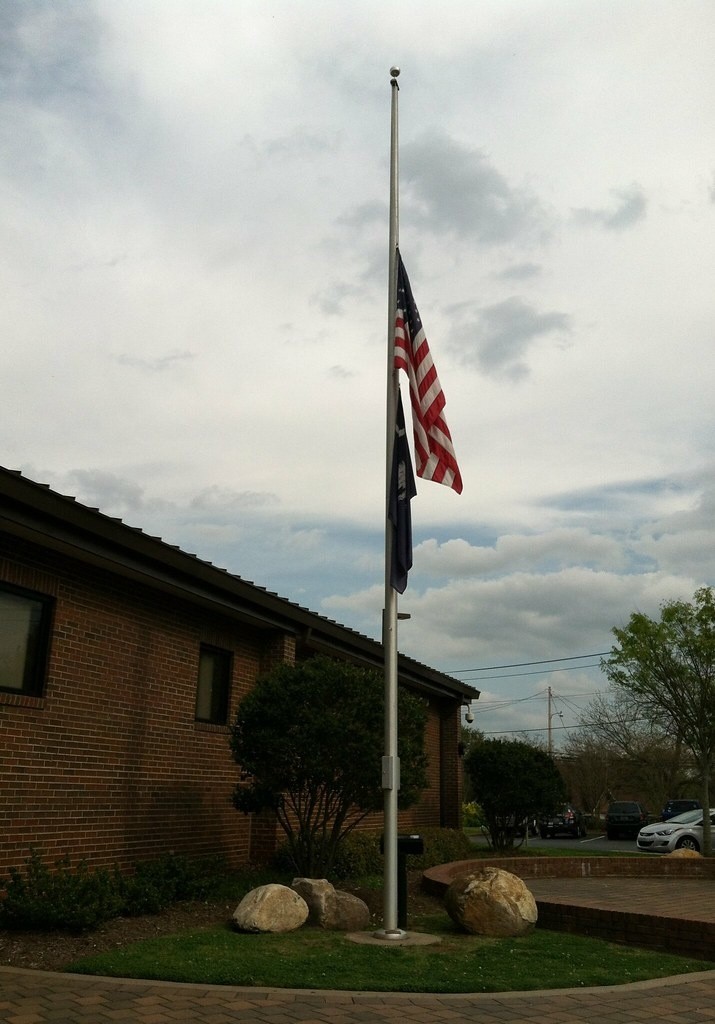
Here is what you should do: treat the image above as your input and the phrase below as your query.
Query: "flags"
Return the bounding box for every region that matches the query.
[386,244,465,602]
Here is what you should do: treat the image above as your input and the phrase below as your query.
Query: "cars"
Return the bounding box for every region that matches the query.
[637,808,714,859]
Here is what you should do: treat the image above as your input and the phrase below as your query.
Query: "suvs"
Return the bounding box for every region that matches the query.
[658,799,701,824]
[605,799,650,839]
[538,802,588,840]
